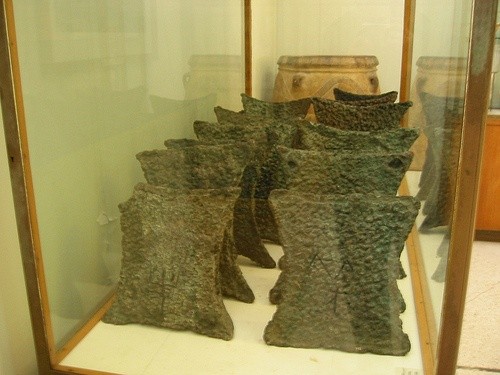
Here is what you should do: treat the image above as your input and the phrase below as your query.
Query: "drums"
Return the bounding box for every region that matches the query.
[409,58,468,172]
[272,56,381,121]
[185,54,247,132]
